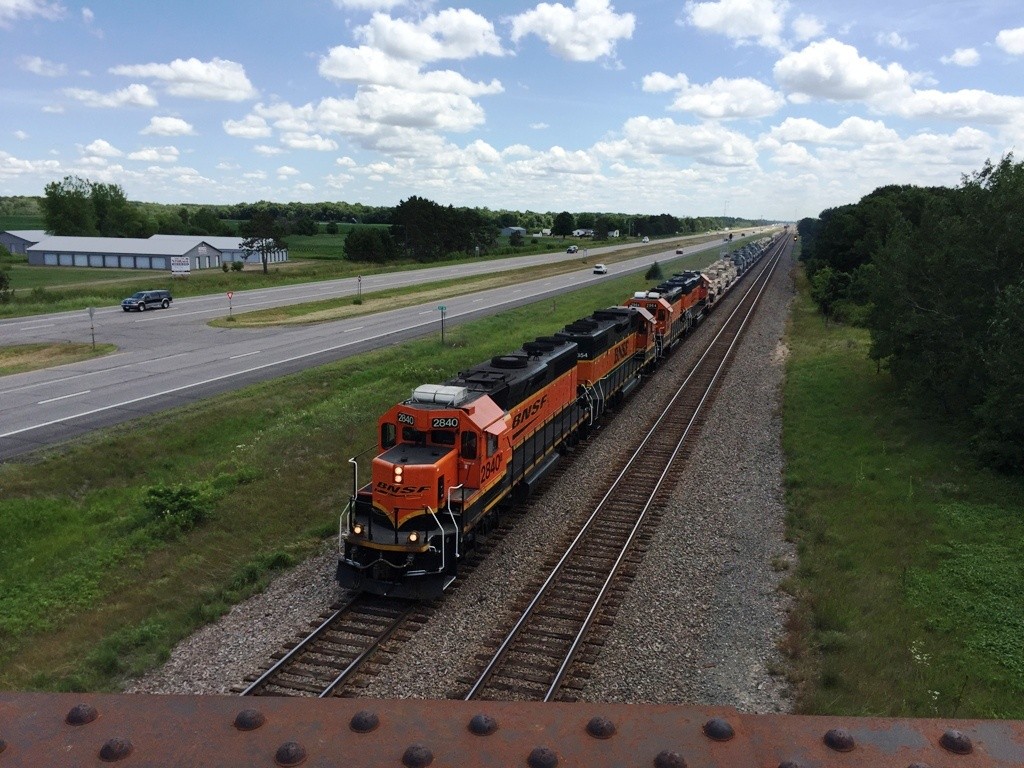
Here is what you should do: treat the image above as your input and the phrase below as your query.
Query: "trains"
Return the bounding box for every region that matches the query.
[336,224,792,609]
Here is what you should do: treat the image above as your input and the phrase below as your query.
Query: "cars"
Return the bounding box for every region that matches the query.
[642,237,649,243]
[122,290,173,312]
[593,264,607,274]
[676,249,683,254]
[567,246,578,254]
